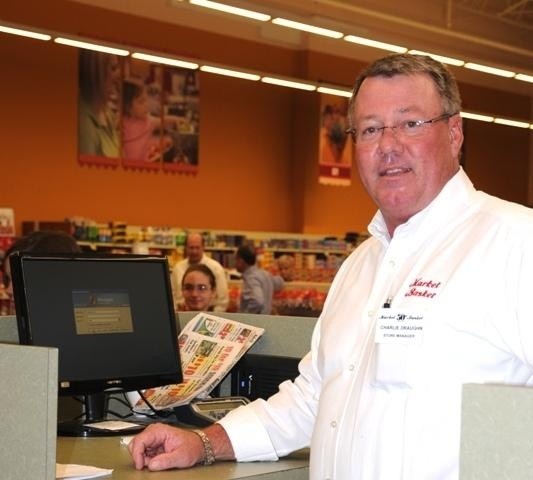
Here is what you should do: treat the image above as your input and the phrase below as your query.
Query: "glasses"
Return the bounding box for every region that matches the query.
[344,112,459,146]
[184,284,208,291]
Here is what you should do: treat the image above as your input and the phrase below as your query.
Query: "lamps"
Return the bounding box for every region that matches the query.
[464,62,516,78]
[408,50,464,67]
[514,73,533,83]
[343,34,408,54]
[272,17,344,39]
[260,73,317,91]
[0,22,54,40]
[199,62,261,81]
[130,46,199,69]
[460,111,494,122]
[316,82,354,97]
[54,32,130,56]
[189,1,271,22]
[494,117,532,130]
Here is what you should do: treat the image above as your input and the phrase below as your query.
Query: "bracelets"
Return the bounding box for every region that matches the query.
[191,429,215,466]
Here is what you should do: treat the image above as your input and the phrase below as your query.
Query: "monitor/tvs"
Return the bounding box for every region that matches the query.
[10,251,184,436]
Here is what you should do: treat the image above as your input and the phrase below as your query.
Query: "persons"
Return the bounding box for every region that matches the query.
[116,78,188,165]
[180,263,217,312]
[0,230,81,318]
[78,48,124,163]
[277,255,295,282]
[318,104,338,166]
[125,54,532,480]
[170,232,230,312]
[234,245,285,373]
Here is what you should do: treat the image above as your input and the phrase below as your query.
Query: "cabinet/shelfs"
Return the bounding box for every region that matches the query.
[76,241,356,316]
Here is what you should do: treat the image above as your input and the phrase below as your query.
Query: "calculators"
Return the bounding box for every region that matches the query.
[188,396,251,428]
